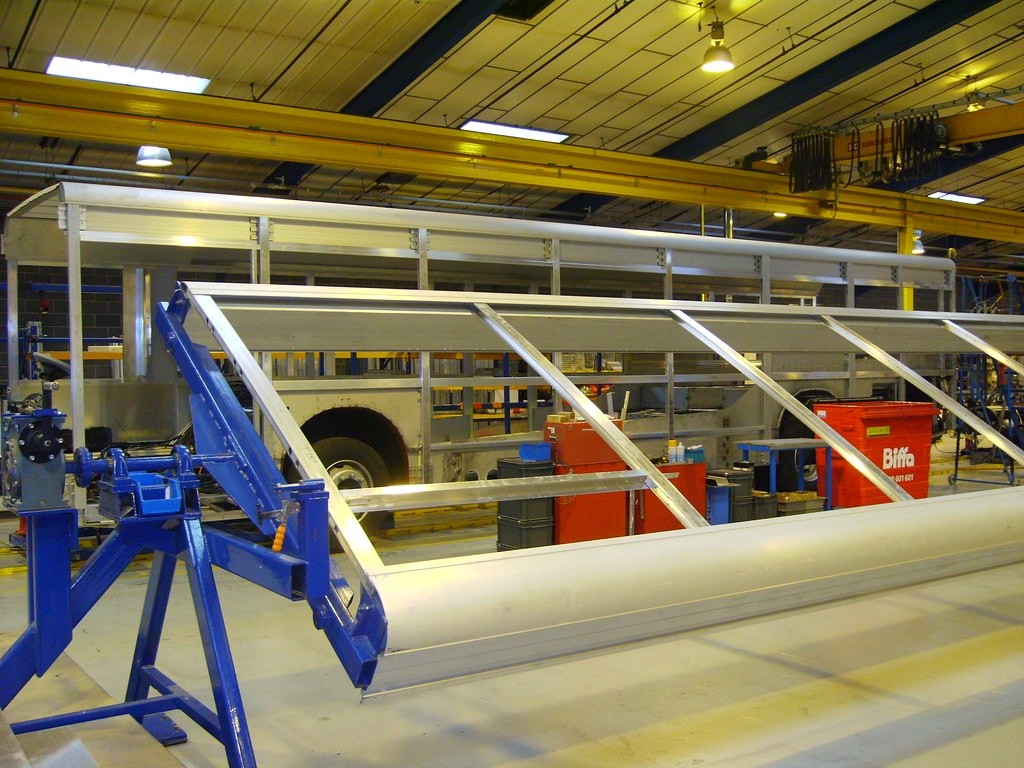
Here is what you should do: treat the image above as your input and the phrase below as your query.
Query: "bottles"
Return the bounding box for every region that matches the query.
[677,442,685,462]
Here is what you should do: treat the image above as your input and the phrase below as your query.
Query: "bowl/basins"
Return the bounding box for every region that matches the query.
[685,449,705,462]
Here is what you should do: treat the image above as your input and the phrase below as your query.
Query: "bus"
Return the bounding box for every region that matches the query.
[0,182,962,556]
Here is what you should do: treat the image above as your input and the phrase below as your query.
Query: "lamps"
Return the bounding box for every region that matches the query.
[700,5,734,73]
[911,229,924,254]
[136,146,173,167]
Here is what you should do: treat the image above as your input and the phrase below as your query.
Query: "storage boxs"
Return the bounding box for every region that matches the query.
[684,448,704,462]
[495,457,557,552]
[706,469,828,526]
[519,442,552,461]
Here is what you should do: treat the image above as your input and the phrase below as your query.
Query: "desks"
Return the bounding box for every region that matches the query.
[734,438,832,511]
